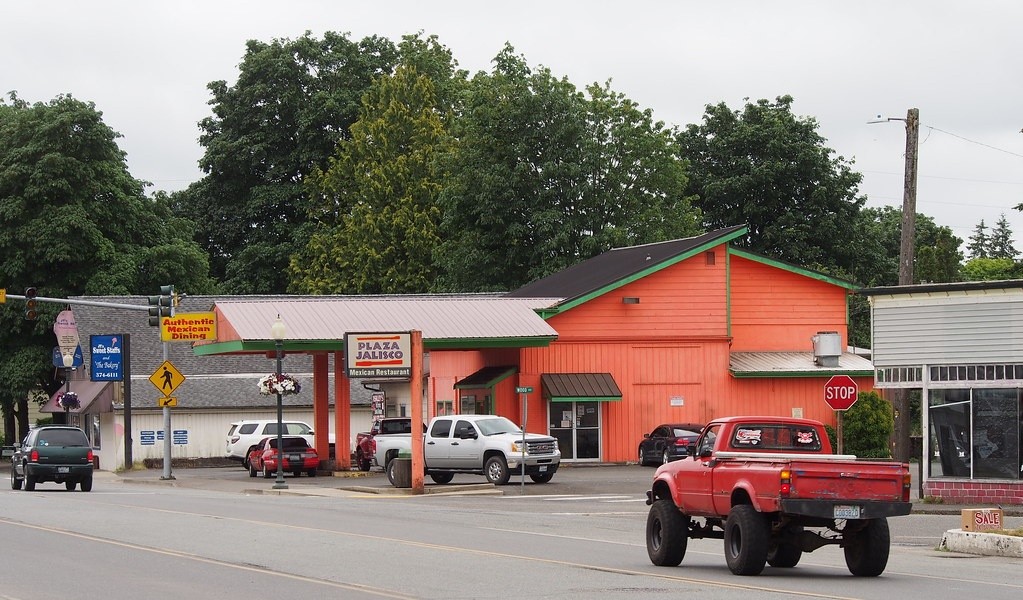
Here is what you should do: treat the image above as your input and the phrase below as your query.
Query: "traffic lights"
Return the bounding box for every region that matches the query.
[147,296,160,328]
[160,285,175,318]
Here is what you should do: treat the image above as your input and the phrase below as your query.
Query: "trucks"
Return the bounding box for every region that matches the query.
[373,414,561,486]
[355,417,428,471]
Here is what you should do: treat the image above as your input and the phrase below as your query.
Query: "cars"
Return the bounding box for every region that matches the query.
[247,435,320,477]
[639,424,716,465]
[225,419,353,470]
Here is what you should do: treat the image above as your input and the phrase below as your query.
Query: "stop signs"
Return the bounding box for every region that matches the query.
[824,375,859,411]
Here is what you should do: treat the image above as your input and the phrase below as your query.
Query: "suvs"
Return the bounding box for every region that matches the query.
[10,426,93,493]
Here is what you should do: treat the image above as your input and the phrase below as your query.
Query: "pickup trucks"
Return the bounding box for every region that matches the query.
[645,415,912,578]
